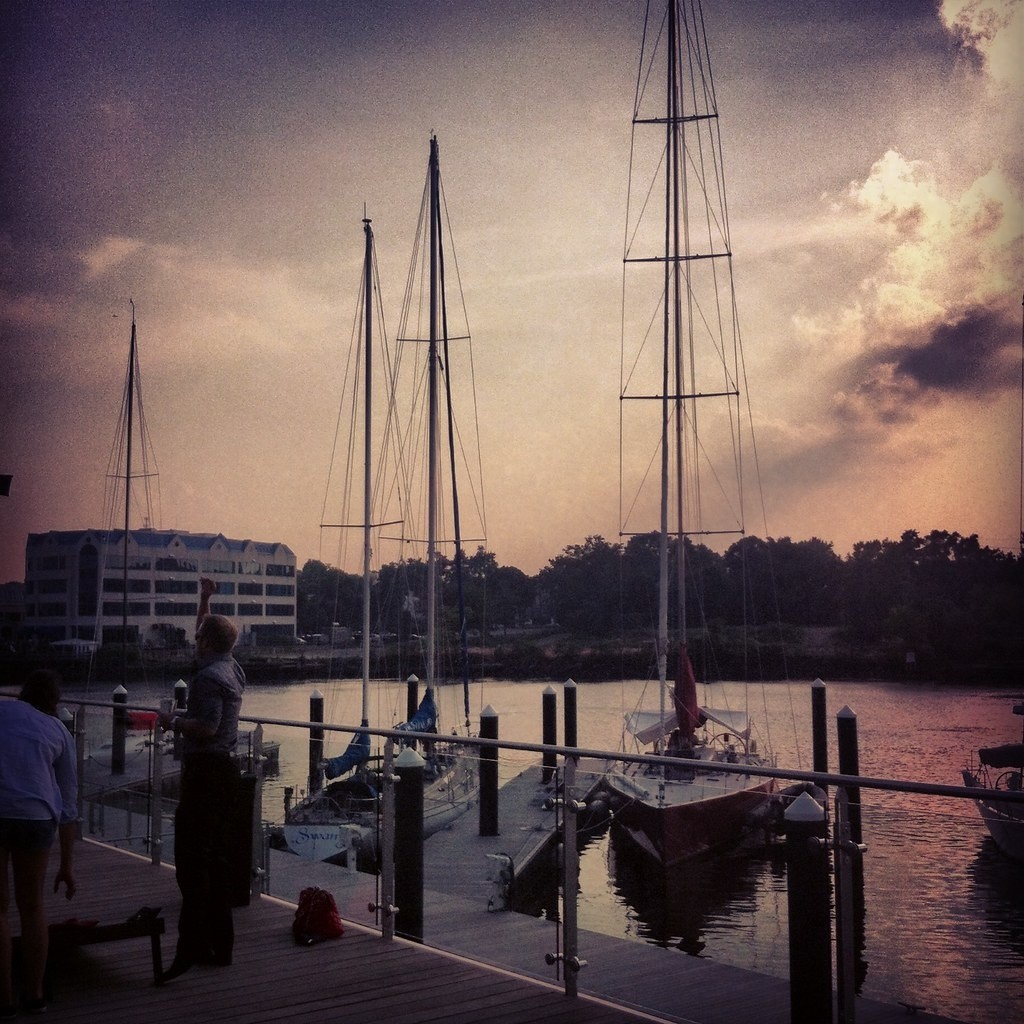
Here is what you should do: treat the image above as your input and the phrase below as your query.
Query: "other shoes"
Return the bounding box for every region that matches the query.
[19,999,47,1014]
[0,1003,17,1019]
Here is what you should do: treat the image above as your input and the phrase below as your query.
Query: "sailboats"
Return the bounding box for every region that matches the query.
[284,128,480,861]
[603,0,783,867]
[75,298,281,805]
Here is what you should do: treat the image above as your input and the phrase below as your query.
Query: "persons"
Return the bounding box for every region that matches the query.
[157,578,245,982]
[0,668,80,1021]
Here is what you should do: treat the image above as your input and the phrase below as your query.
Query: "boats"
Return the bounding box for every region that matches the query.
[960,702,1024,856]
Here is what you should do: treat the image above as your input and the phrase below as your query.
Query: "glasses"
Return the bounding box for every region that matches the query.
[195,632,203,640]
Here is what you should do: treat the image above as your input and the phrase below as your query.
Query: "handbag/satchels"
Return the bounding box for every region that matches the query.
[292,886,345,947]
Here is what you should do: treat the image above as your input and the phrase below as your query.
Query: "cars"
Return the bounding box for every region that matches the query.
[289,631,426,645]
